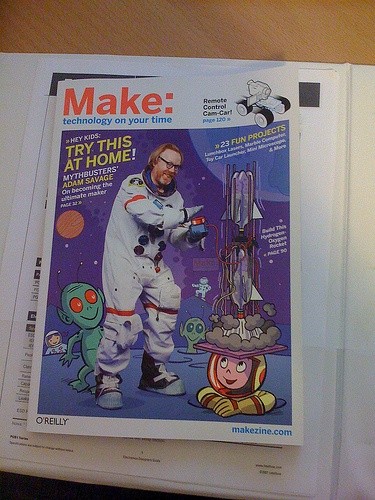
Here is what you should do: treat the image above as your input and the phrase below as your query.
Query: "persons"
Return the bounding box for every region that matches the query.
[94,144,204,409]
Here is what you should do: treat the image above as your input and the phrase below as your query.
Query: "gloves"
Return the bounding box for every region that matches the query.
[189,223,208,242]
[185,205,204,221]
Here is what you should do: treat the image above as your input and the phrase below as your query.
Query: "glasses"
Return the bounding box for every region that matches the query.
[158,156,182,171]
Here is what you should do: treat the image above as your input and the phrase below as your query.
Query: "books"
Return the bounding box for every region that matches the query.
[26,64,302,446]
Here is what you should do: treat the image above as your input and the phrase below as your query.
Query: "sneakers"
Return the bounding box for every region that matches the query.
[139,351,185,395]
[95,368,123,409]
